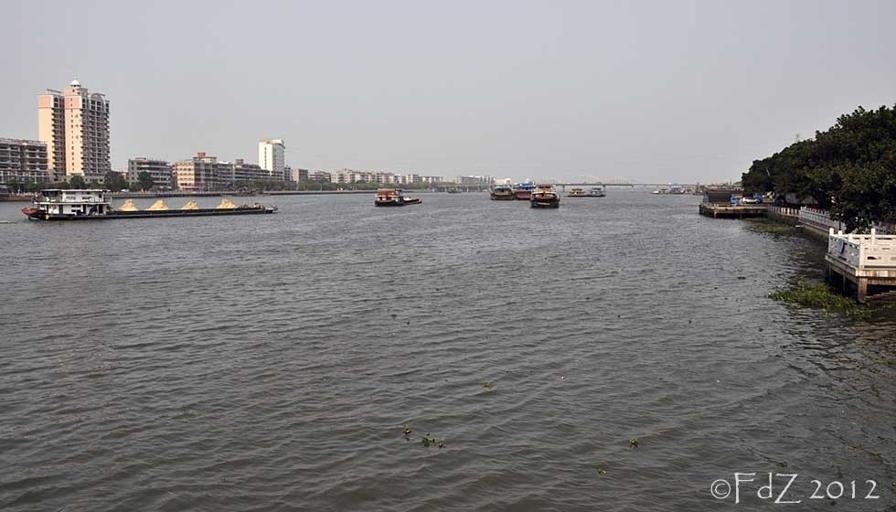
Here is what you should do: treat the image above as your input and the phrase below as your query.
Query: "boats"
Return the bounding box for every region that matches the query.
[528,185,560,208]
[568,185,589,197]
[488,177,513,200]
[649,183,707,196]
[446,187,462,193]
[374,186,421,205]
[22,187,278,222]
[590,183,605,196]
[513,177,535,200]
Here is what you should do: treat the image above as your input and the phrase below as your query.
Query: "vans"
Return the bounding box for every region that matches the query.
[744,192,763,204]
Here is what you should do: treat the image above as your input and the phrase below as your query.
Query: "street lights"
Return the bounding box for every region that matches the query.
[832,192,843,232]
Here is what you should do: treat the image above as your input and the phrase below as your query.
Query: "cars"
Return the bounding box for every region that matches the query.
[743,196,760,205]
